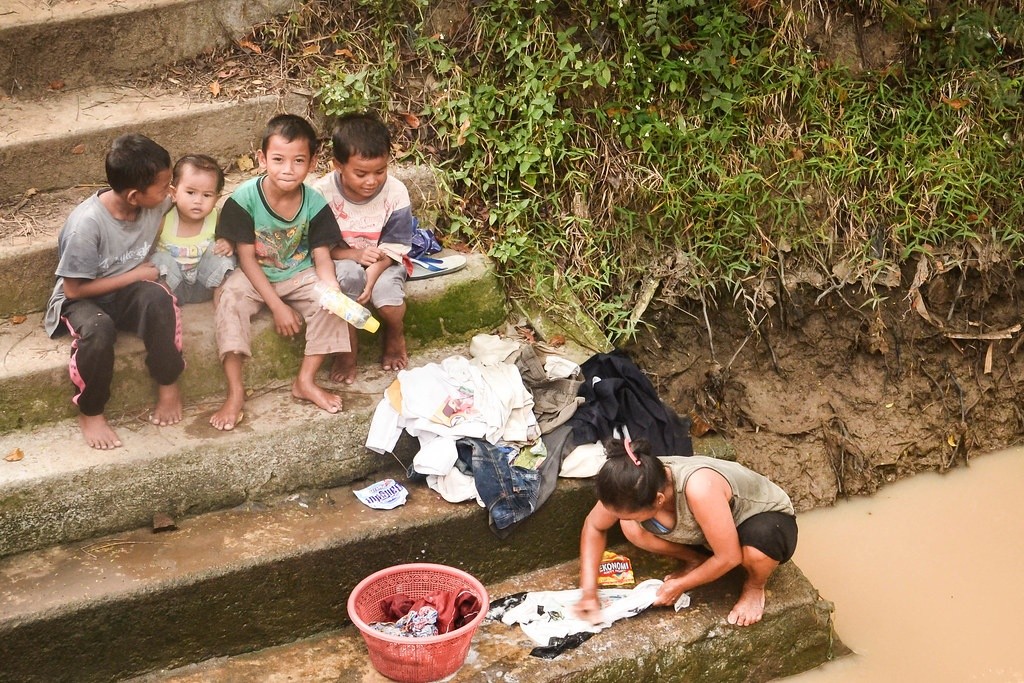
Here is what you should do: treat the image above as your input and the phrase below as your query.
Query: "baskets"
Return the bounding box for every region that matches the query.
[348,563,489,683]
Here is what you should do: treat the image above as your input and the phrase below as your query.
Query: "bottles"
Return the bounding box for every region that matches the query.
[311,280,381,333]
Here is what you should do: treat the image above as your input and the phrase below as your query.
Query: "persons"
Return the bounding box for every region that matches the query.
[571,438,801,627]
[44,115,414,451]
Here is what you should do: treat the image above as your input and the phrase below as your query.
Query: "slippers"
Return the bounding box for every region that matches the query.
[407,254,467,281]
